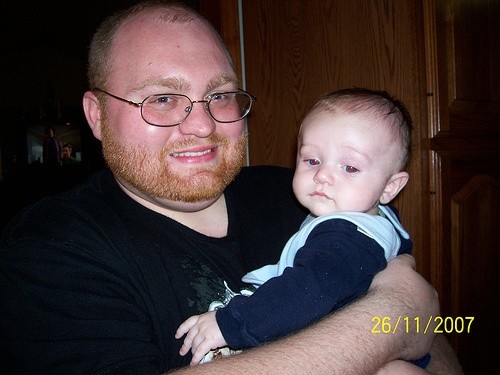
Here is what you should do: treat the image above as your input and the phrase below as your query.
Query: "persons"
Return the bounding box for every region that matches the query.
[174,86,433,373]
[0,0,465,375]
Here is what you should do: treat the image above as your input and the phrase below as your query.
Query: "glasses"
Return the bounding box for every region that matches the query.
[94,88,257,127]
